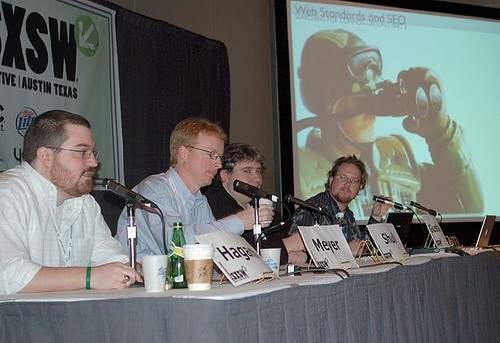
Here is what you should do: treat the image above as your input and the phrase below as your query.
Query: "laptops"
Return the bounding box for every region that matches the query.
[462,215,496,250]
[358,211,414,257]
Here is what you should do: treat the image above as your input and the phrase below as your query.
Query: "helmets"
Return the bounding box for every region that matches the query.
[300,29,384,145]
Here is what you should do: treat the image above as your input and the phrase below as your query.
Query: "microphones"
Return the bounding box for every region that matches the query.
[283,195,329,215]
[410,201,438,216]
[372,195,410,210]
[232,180,279,202]
[101,177,157,209]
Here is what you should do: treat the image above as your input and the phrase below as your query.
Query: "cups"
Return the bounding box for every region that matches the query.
[141,255,167,292]
[260,248,281,276]
[183,244,213,290]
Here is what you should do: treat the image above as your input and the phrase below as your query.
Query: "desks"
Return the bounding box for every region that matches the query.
[0,242,500,343]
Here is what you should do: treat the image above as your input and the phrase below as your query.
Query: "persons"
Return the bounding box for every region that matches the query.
[289,154,393,256]
[201,142,312,264]
[295,29,485,216]
[0,109,144,295]
[114,117,275,264]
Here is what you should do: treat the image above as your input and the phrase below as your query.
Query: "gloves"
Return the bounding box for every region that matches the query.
[395,67,449,141]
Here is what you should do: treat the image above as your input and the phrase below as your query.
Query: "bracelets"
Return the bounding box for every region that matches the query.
[86,267,91,289]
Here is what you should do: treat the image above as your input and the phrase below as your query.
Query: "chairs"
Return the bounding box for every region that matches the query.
[90,190,125,238]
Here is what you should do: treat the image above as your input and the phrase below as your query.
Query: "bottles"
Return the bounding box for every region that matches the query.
[168,222,187,289]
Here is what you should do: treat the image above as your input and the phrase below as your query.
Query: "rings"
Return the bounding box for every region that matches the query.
[123,275,130,283]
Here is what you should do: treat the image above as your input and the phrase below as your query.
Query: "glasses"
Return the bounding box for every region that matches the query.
[184,146,223,160]
[343,46,383,79]
[333,174,362,186]
[43,146,99,159]
